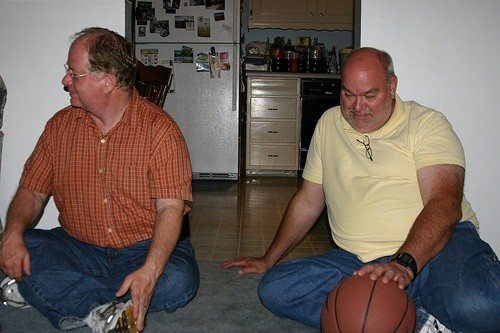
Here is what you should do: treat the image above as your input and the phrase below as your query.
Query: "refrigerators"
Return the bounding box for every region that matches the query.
[133,0,244,181]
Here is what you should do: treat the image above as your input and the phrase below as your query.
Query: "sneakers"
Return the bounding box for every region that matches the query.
[83,298,139,333]
[0,276,31,309]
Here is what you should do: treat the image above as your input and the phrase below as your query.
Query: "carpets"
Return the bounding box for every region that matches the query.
[0,259,321,333]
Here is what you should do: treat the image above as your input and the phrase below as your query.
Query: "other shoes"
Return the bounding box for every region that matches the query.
[419,314,454,333]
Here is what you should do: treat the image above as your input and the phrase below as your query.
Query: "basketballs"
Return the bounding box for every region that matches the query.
[320,273,418,333]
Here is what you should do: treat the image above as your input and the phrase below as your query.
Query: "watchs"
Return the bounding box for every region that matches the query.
[390,252,417,281]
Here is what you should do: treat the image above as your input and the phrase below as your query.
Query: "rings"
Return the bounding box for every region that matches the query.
[145,306,147,309]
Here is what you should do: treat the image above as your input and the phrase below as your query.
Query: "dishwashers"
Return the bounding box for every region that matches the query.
[299,79,341,173]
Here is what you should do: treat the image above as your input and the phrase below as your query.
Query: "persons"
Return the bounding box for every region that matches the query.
[222,47,500,333]
[0,27,200,333]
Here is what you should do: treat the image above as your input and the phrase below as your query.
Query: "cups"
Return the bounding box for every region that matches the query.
[339,48,353,73]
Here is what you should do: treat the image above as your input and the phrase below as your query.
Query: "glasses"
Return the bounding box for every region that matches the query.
[64,62,101,81]
[356,135,373,161]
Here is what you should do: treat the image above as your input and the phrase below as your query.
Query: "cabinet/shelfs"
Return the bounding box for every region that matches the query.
[245,69,301,180]
[247,0,354,32]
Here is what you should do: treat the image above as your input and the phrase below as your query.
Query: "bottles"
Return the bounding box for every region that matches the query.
[268,36,337,74]
